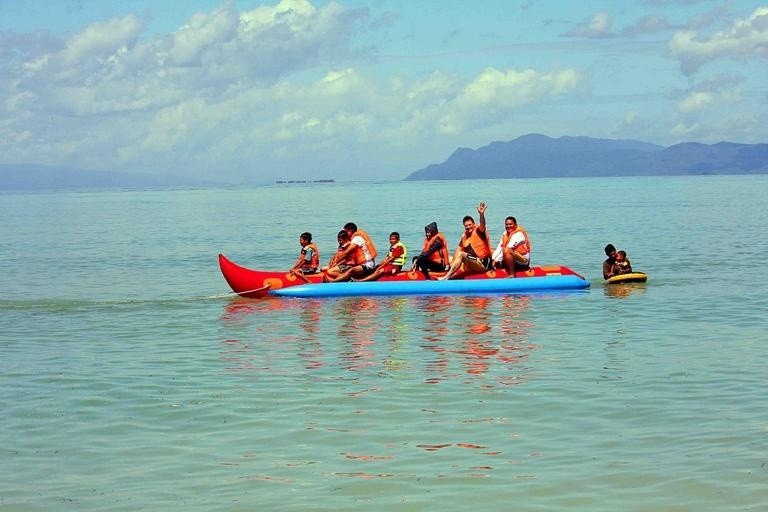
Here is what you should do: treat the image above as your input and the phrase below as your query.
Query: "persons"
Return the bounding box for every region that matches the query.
[320,223,377,283]
[436,202,493,280]
[492,216,530,278]
[289,232,319,283]
[409,222,451,280]
[603,244,632,280]
[351,232,407,281]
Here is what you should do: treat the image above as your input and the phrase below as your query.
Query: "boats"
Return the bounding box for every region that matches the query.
[219,253,590,300]
[602,271,648,287]
